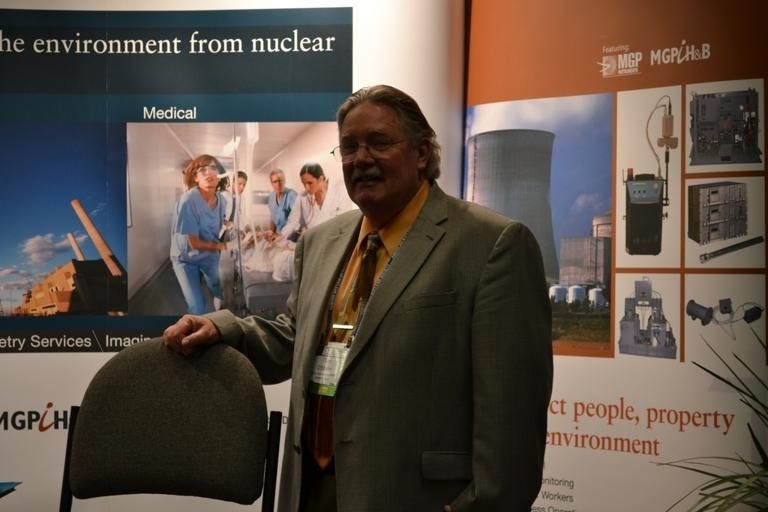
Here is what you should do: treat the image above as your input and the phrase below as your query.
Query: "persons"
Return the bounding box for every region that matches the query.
[162,88,553,512]
[170,154,352,318]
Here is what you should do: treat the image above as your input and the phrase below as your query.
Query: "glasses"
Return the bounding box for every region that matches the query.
[330,136,421,163]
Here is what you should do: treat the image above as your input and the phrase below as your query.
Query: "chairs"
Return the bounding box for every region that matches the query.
[57,335,284,512]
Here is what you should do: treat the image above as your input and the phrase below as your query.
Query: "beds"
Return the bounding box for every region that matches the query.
[233,241,295,319]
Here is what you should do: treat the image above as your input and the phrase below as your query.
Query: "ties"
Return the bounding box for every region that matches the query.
[310,231,385,470]
